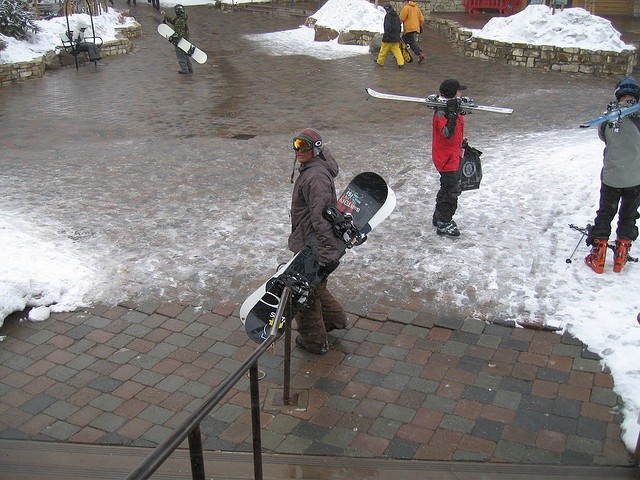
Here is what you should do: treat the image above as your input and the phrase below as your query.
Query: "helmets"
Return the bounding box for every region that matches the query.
[174,5,184,12]
[614,76,640,101]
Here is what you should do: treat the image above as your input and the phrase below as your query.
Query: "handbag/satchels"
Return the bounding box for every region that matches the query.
[459,145,482,191]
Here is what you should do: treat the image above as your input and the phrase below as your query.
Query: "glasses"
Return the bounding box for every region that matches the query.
[293,138,312,152]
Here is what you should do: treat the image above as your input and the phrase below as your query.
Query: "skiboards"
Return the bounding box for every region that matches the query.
[366,87,513,114]
[580,101,639,132]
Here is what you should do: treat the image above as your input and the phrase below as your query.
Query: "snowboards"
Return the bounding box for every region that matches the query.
[157,23,208,65]
[246,171,387,345]
[398,38,413,63]
[239,179,396,325]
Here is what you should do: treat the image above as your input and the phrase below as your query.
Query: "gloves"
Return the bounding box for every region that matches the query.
[317,262,337,277]
[447,99,461,111]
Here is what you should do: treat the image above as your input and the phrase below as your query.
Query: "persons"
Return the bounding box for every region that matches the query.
[432,78,467,237]
[162,4,194,74]
[125,1,137,5]
[72,20,104,63]
[400,2,425,64]
[373,4,405,68]
[584,77,639,274]
[287,129,351,355]
[149,0,160,10]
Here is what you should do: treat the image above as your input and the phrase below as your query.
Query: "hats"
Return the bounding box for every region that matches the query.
[439,78,467,98]
[296,127,322,152]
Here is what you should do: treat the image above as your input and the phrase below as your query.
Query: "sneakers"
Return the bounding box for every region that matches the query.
[613,239,633,273]
[328,317,350,332]
[295,334,329,354]
[418,52,426,63]
[91,57,102,62]
[432,218,460,236]
[584,239,608,274]
